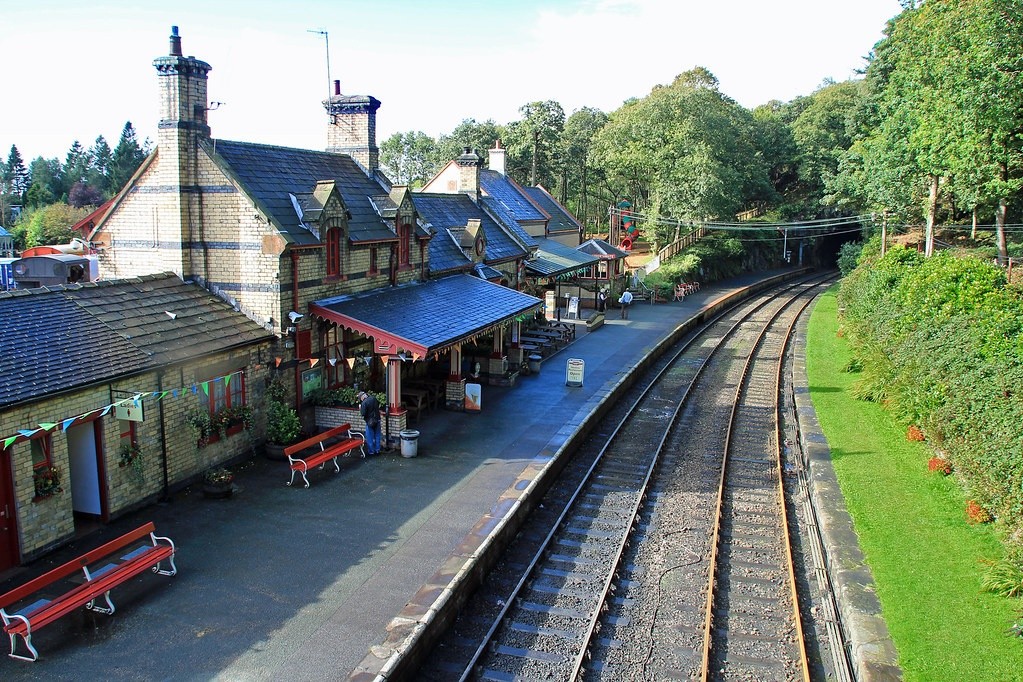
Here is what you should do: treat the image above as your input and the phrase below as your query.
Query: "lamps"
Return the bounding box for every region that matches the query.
[288,312,303,323]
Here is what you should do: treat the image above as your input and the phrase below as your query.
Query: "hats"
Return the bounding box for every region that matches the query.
[600,288,605,292]
[358,391,364,401]
[626,288,629,290]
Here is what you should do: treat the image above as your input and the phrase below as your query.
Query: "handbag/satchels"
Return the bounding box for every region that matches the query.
[367,416,379,428]
[618,297,625,303]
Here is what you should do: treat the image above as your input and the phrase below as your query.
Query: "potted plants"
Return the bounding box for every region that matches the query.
[263,401,303,461]
[192,406,257,457]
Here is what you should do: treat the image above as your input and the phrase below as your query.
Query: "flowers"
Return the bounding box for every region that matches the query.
[34,467,65,496]
[204,467,233,487]
[268,377,290,401]
[119,441,144,467]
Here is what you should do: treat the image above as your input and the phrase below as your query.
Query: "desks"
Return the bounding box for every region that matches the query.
[505,320,575,356]
[410,379,443,409]
[402,388,430,424]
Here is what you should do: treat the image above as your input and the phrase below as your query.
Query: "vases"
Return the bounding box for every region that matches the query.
[204,481,233,498]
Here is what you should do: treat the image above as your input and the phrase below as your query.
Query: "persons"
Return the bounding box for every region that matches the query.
[599,288,607,312]
[358,391,380,455]
[619,288,633,319]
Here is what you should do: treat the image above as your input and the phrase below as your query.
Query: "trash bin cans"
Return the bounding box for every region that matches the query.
[528,354,542,373]
[400,429,420,458]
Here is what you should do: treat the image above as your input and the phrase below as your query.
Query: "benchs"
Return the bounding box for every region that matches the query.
[285,424,366,489]
[504,329,575,360]
[0,521,176,663]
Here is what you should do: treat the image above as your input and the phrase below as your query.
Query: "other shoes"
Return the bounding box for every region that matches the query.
[375,453,380,456]
[368,454,374,457]
